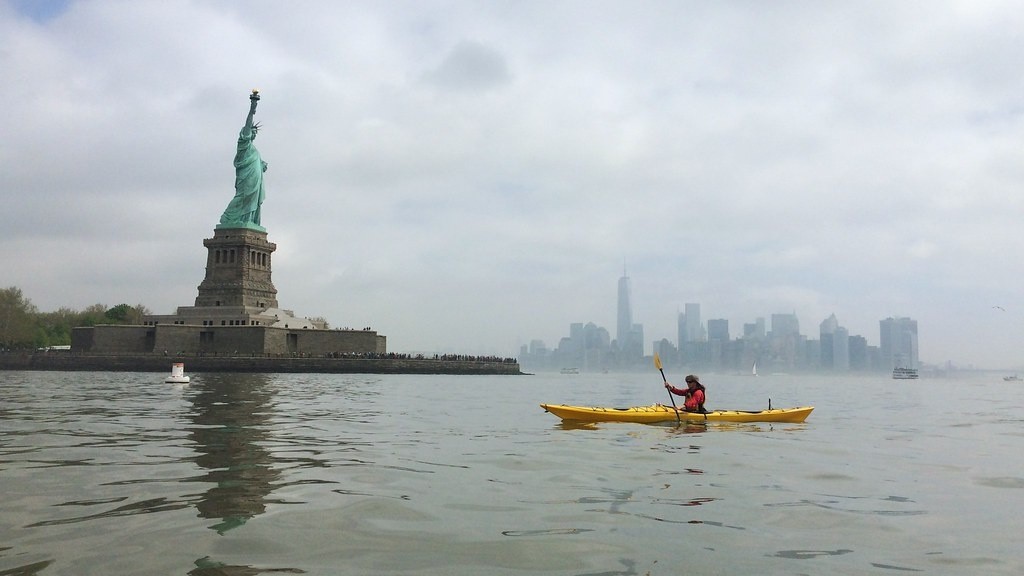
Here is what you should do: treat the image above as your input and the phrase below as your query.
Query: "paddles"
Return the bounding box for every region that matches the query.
[654,352,681,423]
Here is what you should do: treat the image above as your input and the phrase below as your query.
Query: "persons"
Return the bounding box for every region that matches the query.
[163,347,518,363]
[335,325,374,331]
[220,99,267,225]
[664,375,706,412]
[33,346,59,354]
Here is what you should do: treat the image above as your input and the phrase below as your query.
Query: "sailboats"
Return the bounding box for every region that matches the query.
[752,361,759,377]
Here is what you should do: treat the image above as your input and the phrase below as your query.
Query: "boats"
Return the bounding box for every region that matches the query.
[893,364,920,379]
[164,363,191,384]
[1004,375,1023,382]
[538,398,816,424]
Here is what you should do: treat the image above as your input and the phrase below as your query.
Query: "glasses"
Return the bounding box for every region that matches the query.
[686,380,694,383]
